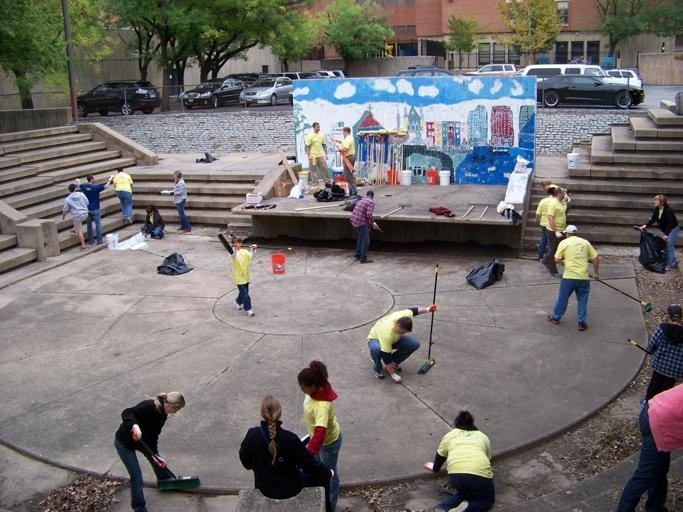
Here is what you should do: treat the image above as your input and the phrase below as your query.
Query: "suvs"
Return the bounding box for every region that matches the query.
[462,64,517,77]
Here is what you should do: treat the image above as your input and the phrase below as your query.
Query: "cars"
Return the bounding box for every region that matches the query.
[223,70,350,87]
[395,65,458,77]
[77,80,161,118]
[183,78,249,110]
[537,74,645,109]
[238,76,292,108]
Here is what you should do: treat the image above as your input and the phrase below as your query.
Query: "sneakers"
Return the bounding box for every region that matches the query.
[361,258,372,263]
[244,309,254,317]
[391,365,402,373]
[447,501,468,512]
[577,322,588,331]
[545,314,560,326]
[176,226,191,235]
[372,368,386,380]
[233,300,242,310]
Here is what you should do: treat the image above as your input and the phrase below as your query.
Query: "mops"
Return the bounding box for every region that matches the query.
[587,274,654,313]
[417,263,440,376]
[380,202,411,220]
[462,202,497,219]
[355,129,409,185]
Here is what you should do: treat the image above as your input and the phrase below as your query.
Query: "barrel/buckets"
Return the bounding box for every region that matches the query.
[426,169,438,185]
[387,169,412,185]
[566,153,580,169]
[298,171,309,185]
[271,253,286,274]
[105,233,119,250]
[332,170,344,184]
[438,170,451,186]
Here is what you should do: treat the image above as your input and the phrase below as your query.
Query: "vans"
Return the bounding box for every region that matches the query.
[514,64,642,88]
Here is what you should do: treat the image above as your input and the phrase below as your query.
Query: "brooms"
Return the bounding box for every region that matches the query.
[130,431,200,491]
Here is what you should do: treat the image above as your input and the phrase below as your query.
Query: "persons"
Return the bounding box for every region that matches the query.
[646,304,683,401]
[239,395,335,512]
[350,191,379,263]
[545,186,567,276]
[75,175,114,245]
[423,410,495,512]
[304,122,330,190]
[535,183,558,259]
[333,127,361,199]
[297,360,342,511]
[615,383,683,512]
[639,194,680,271]
[140,204,165,239]
[169,170,192,234]
[545,224,599,331]
[367,304,438,379]
[229,236,258,317]
[112,167,134,225]
[113,391,187,512]
[62,184,89,251]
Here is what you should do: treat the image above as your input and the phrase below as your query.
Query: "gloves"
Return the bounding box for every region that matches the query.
[423,461,433,472]
[150,454,167,470]
[129,423,143,442]
[425,304,439,314]
[390,373,401,384]
[554,231,564,238]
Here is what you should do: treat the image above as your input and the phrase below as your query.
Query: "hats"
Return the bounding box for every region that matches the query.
[668,303,682,317]
[561,224,577,235]
